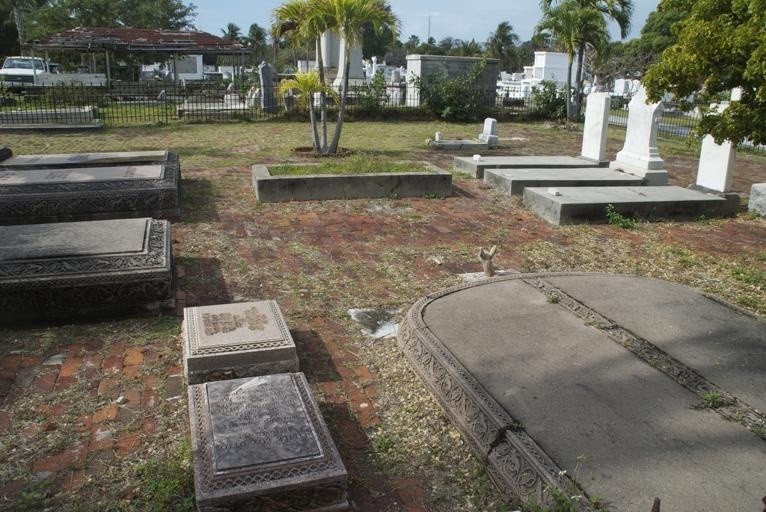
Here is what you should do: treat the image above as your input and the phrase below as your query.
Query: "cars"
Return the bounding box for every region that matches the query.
[47,63,64,74]
[0,55,49,92]
